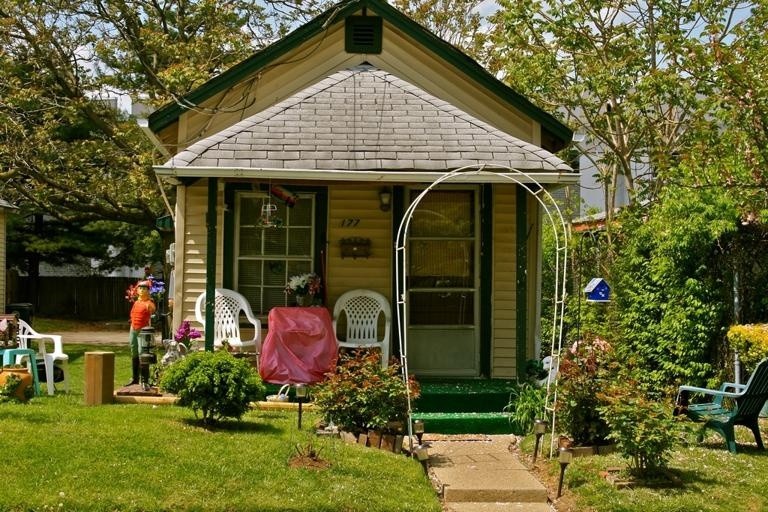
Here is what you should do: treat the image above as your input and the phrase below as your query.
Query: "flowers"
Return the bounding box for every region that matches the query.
[282,271,321,298]
[173,319,202,354]
[124,275,165,303]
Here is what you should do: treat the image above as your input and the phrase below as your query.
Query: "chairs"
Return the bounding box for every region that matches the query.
[193,287,262,375]
[330,287,393,372]
[670,357,768,455]
[14,318,70,397]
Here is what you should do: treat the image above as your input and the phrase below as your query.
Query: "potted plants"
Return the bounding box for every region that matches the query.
[0,362,33,401]
[314,343,423,454]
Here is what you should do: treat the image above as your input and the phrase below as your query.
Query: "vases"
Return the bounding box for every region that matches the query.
[296,294,316,307]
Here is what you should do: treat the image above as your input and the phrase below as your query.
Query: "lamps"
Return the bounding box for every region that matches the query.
[380,186,392,212]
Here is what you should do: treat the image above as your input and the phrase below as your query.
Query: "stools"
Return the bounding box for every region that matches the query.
[0,348,41,398]
